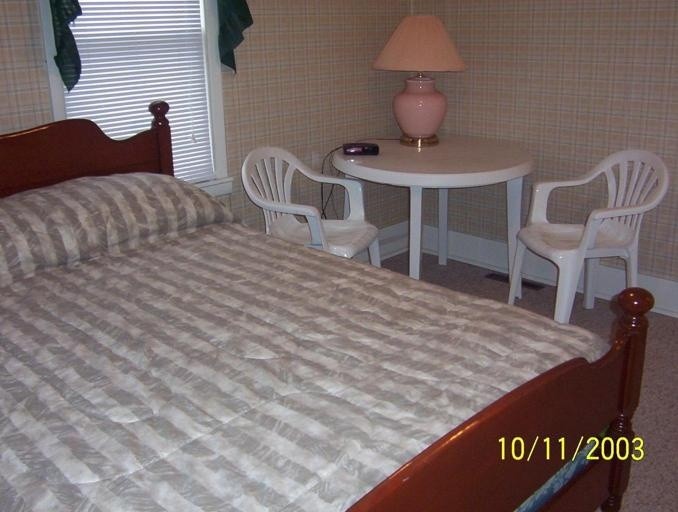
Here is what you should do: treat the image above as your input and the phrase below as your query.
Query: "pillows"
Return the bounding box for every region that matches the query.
[1,171,238,292]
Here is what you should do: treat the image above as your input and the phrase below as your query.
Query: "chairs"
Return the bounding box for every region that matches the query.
[240,146,382,268]
[505,148,669,326]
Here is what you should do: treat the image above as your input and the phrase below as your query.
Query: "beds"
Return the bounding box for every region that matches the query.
[0,99,656,511]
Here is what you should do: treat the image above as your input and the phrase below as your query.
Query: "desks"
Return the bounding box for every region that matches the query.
[330,134,534,301]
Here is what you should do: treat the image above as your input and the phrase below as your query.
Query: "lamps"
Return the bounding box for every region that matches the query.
[369,15,468,149]
